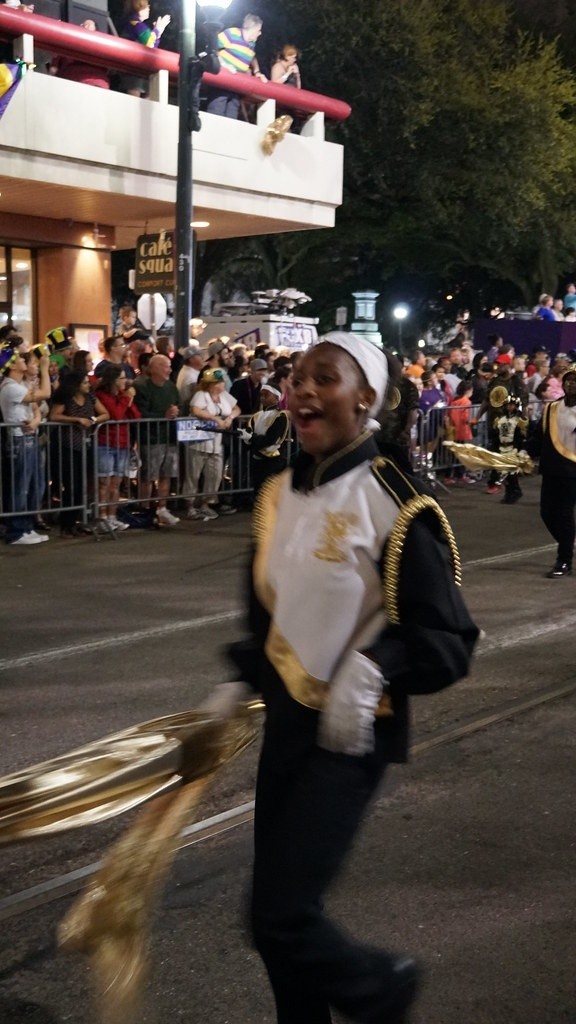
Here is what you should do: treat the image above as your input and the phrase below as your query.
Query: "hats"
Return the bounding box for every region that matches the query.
[494,353,512,364]
[45,327,76,356]
[0,342,17,373]
[250,359,271,374]
[183,346,206,360]
[202,368,227,384]
[27,343,50,366]
[206,341,227,363]
[124,327,153,344]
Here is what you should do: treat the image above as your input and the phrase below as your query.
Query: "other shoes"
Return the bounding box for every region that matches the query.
[357,949,420,1024]
[98,517,118,532]
[29,530,49,542]
[216,504,236,515]
[548,560,572,578]
[486,484,501,495]
[459,474,476,484]
[155,506,179,524]
[110,515,129,530]
[201,507,218,519]
[12,532,42,545]
[442,477,456,485]
[184,507,209,522]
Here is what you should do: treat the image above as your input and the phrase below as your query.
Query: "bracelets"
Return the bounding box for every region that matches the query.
[472,418,477,423]
[254,71,260,75]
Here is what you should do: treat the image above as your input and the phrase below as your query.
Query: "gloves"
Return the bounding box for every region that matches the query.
[196,680,250,726]
[320,650,384,756]
[236,427,252,445]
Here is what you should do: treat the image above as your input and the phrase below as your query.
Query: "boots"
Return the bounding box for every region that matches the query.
[503,477,524,504]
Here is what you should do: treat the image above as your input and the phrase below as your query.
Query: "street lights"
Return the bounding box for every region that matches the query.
[168,1,234,357]
[393,305,408,359]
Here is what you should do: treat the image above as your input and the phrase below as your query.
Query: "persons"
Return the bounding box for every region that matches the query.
[0,306,306,544]
[529,370,576,578]
[0,0,34,65]
[272,45,302,89]
[392,323,576,494]
[208,331,481,1024]
[50,19,111,90]
[116,0,171,99]
[531,283,576,322]
[469,354,529,504]
[207,13,267,120]
